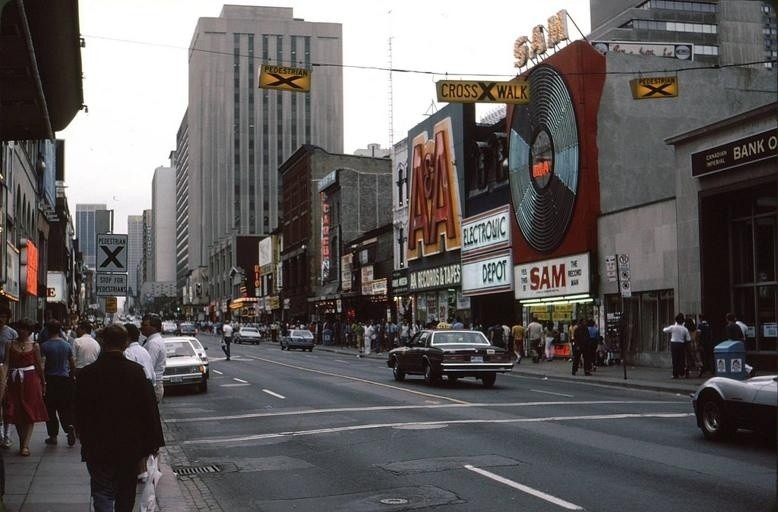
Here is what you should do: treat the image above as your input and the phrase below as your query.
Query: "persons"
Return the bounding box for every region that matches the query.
[663,314,755,379]
[319,316,462,355]
[0,306,168,512]
[174,317,319,342]
[468,316,558,363]
[222,320,233,361]
[568,319,611,376]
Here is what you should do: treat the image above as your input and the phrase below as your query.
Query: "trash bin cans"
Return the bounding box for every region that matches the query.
[712,340,745,380]
[322,330,333,345]
[272,329,277,342]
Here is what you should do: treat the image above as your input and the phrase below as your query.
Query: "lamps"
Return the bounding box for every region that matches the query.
[78,38,86,48]
[80,103,89,115]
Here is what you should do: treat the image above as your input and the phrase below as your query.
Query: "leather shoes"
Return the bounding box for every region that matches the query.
[21,446,29,457]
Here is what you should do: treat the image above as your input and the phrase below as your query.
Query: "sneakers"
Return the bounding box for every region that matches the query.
[66,423,76,448]
[2,439,16,450]
[45,438,58,446]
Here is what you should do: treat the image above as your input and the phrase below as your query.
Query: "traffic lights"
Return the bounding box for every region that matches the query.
[177,307,181,315]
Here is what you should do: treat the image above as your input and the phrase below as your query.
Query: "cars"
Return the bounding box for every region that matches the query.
[116,312,143,322]
[233,326,262,345]
[280,329,316,352]
[164,335,209,379]
[383,321,516,389]
[162,338,208,394]
[177,321,198,336]
[160,321,178,335]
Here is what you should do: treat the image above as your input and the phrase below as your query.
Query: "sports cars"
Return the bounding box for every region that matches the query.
[689,373,777,441]
